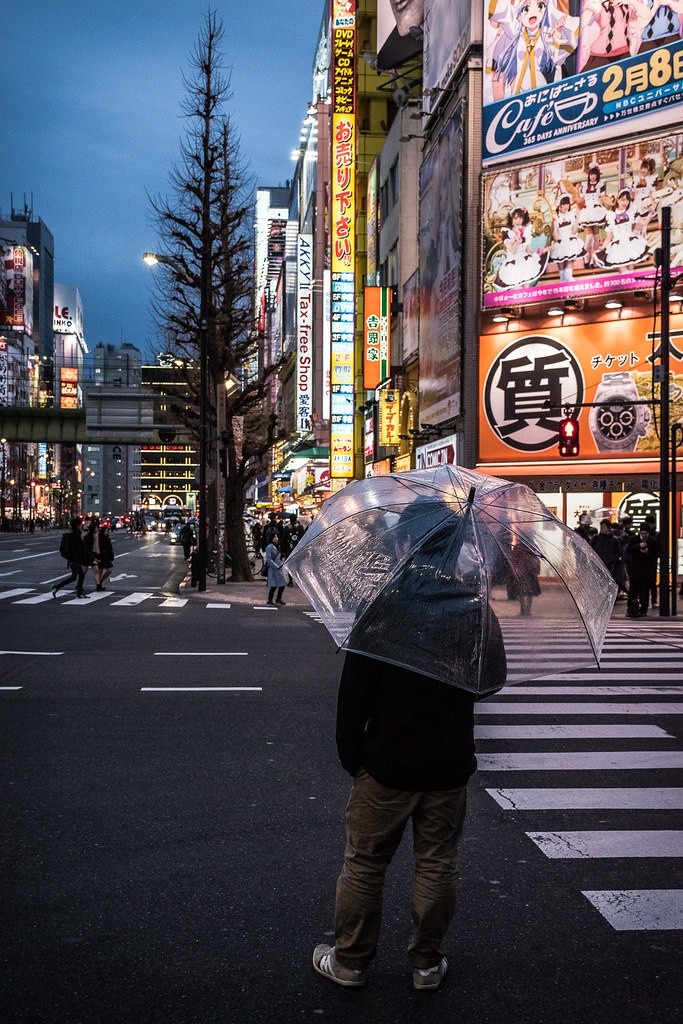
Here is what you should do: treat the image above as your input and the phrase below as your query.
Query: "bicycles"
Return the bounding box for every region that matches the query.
[205,542,265,578]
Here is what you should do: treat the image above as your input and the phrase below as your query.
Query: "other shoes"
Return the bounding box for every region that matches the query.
[288,582,293,586]
[266,601,278,607]
[626,612,631,617]
[652,603,659,609]
[96,583,106,591]
[640,613,646,617]
[275,600,286,605]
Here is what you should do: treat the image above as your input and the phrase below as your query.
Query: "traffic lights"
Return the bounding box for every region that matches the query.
[558,418,581,457]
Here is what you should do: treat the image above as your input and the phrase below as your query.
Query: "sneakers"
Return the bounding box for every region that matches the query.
[313,944,365,986]
[76,595,90,598]
[413,957,448,988]
[53,584,59,599]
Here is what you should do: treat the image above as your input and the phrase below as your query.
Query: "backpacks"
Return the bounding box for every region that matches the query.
[266,524,279,542]
[59,533,74,558]
[288,524,302,549]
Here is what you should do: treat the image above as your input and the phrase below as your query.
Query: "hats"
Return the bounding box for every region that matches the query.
[640,522,650,531]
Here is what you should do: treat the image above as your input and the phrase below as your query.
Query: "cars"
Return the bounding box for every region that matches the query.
[171,523,196,545]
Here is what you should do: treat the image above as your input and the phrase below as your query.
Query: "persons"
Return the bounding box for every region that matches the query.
[313,495,507,991]
[0,508,194,603]
[574,513,660,618]
[457,512,541,614]
[491,157,658,290]
[242,509,326,608]
[390,0,424,37]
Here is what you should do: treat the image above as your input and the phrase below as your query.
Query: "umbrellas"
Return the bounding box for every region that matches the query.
[581,506,630,524]
[277,462,622,701]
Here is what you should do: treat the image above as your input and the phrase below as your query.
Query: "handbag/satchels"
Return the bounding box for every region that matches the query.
[629,599,639,618]
[261,566,268,576]
